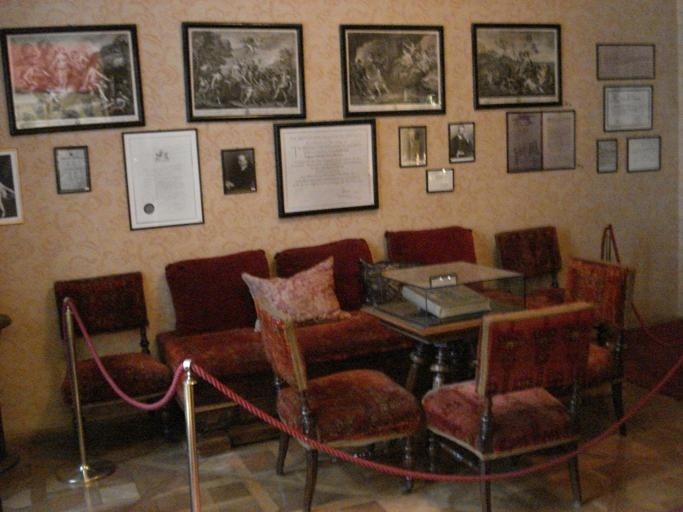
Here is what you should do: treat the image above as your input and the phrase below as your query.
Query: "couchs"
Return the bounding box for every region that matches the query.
[157,288,541,455]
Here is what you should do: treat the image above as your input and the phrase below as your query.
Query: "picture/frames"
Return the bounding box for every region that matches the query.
[596,42,656,81]
[339,24,445,117]
[471,23,562,109]
[0,24,146,136]
[181,21,305,122]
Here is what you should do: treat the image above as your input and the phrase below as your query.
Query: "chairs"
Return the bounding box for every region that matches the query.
[495,226,564,311]
[544,256,635,437]
[422,301,596,512]
[54,271,174,448]
[255,298,422,512]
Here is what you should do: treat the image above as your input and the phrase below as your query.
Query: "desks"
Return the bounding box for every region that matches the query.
[367,262,527,396]
